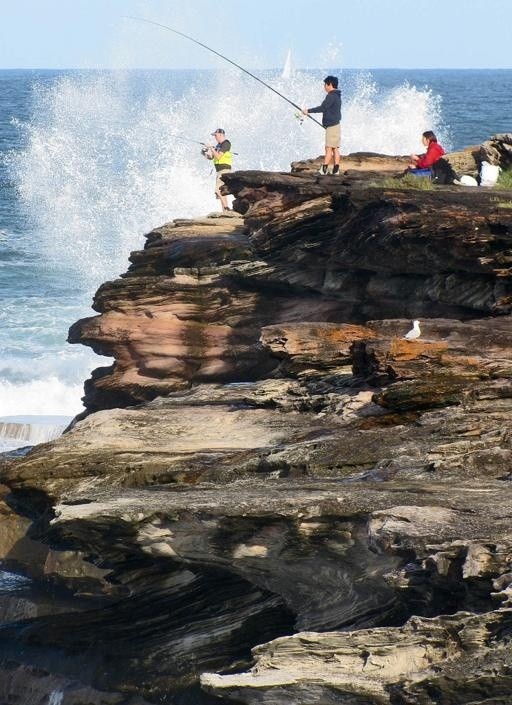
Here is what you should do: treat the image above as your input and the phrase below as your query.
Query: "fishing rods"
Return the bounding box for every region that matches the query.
[109,115,238,155]
[121,14,326,127]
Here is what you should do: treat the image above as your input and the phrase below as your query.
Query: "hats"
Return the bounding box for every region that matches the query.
[210,129,225,134]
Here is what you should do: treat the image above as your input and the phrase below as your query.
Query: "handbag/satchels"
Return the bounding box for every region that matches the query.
[479,161,502,187]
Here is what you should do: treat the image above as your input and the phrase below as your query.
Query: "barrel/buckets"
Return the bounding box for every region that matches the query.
[479,166,499,185]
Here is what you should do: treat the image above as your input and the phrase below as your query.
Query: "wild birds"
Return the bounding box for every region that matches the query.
[402,321,420,339]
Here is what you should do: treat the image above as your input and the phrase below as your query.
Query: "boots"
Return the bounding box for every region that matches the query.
[313,164,340,177]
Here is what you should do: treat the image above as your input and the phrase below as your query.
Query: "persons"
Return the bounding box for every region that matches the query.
[410,130,446,168]
[302,76,342,176]
[201,128,232,213]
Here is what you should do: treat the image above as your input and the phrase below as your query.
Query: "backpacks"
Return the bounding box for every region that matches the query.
[432,158,456,185]
